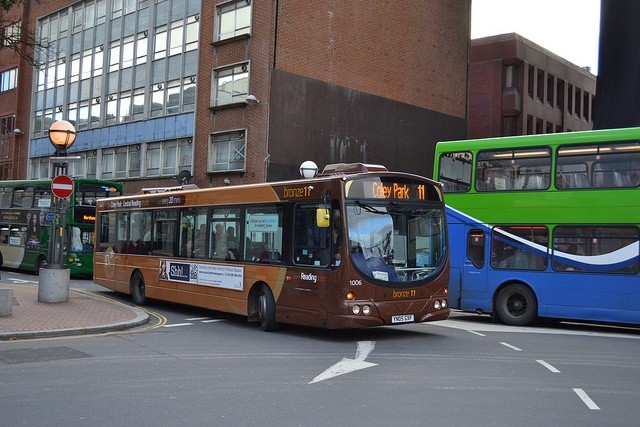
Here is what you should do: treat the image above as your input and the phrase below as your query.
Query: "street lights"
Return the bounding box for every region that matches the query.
[38,120,78,304]
[299,161,319,179]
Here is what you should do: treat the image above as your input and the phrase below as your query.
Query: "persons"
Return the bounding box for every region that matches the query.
[160,262,168,279]
[628,174,639,187]
[225,227,236,259]
[197,224,206,254]
[327,226,343,263]
[39,211,45,226]
[24,211,32,223]
[132,214,142,241]
[564,243,581,271]
[29,212,40,240]
[143,226,148,241]
[212,224,225,255]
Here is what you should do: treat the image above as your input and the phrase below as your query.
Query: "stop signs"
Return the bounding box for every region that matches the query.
[51,175,74,199]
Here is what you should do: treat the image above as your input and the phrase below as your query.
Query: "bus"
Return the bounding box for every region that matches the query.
[92,163,450,332]
[0,178,124,280]
[433,127,640,326]
